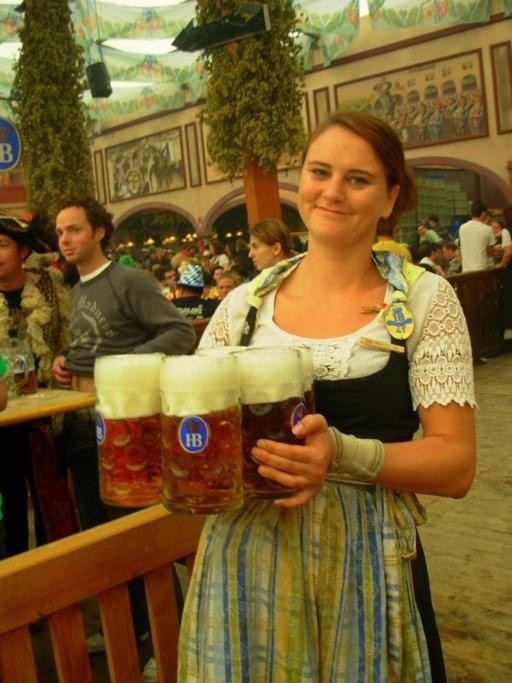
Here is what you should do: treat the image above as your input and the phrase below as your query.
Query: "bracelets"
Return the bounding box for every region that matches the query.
[330,424,387,485]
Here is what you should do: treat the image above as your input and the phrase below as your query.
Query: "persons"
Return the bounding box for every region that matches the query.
[0,213,70,638]
[22,201,512,392]
[49,192,203,682]
[374,75,488,146]
[112,137,173,200]
[174,104,479,682]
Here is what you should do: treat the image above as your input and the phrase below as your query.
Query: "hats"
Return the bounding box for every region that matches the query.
[0,214,51,253]
[178,263,205,287]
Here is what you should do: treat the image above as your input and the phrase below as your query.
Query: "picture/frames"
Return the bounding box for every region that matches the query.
[104,125,186,203]
[184,120,202,188]
[333,47,490,151]
[199,91,312,186]
[311,86,332,129]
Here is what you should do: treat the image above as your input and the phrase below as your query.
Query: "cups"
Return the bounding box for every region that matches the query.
[240,344,318,498]
[10,340,40,395]
[90,352,165,511]
[156,352,243,515]
[0,347,29,412]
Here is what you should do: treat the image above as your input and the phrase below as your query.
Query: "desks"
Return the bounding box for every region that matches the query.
[0,389,99,546]
